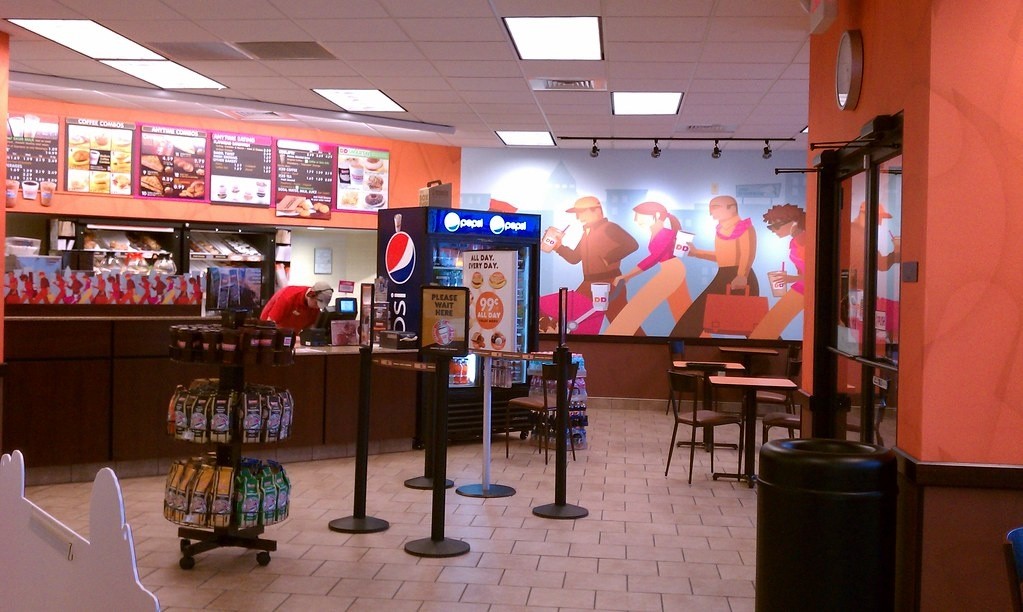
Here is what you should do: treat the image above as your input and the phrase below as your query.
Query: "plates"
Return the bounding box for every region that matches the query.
[364,194,384,207]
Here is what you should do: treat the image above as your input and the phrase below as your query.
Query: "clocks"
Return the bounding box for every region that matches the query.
[835,28,864,112]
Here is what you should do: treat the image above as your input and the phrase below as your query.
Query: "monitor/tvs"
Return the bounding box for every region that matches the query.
[316,306,335,329]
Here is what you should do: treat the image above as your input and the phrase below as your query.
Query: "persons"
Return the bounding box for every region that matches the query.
[259,281,333,336]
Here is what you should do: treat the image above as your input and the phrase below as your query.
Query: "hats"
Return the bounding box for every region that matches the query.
[313,282,333,309]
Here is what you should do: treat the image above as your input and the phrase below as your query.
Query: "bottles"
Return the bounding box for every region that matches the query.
[432,246,462,287]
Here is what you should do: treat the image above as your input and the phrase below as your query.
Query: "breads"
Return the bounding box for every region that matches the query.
[83,231,218,254]
[297,201,329,216]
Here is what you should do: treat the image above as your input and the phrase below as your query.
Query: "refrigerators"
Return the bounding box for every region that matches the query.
[376,209,542,441]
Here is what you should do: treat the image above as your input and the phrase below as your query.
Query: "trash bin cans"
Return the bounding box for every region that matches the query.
[753,436,895,612]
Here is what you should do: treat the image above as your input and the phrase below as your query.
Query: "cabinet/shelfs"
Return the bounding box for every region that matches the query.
[161,304,296,571]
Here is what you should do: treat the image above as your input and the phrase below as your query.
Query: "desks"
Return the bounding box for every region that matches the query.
[707,375,797,489]
[673,359,745,453]
[717,346,779,374]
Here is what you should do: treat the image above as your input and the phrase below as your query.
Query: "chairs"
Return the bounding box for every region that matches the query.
[755,360,802,439]
[847,377,892,446]
[666,369,743,485]
[756,343,801,414]
[505,361,580,466]
[761,412,800,444]
[664,339,704,415]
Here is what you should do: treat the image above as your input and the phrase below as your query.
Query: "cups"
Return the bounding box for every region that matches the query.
[22,181,39,199]
[6,179,20,207]
[339,167,350,187]
[41,182,56,206]
[24,115,40,138]
[90,151,99,164]
[169,320,293,366]
[349,166,363,185]
[9,117,24,138]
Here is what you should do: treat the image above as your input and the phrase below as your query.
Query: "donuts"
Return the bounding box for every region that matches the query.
[366,193,383,205]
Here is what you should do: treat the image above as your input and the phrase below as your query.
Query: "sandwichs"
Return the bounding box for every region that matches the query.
[141,154,163,172]
[140,175,163,194]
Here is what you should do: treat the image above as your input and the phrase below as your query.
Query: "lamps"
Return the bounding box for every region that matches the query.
[762,147,773,159]
[711,147,722,159]
[590,145,600,158]
[651,146,661,159]
